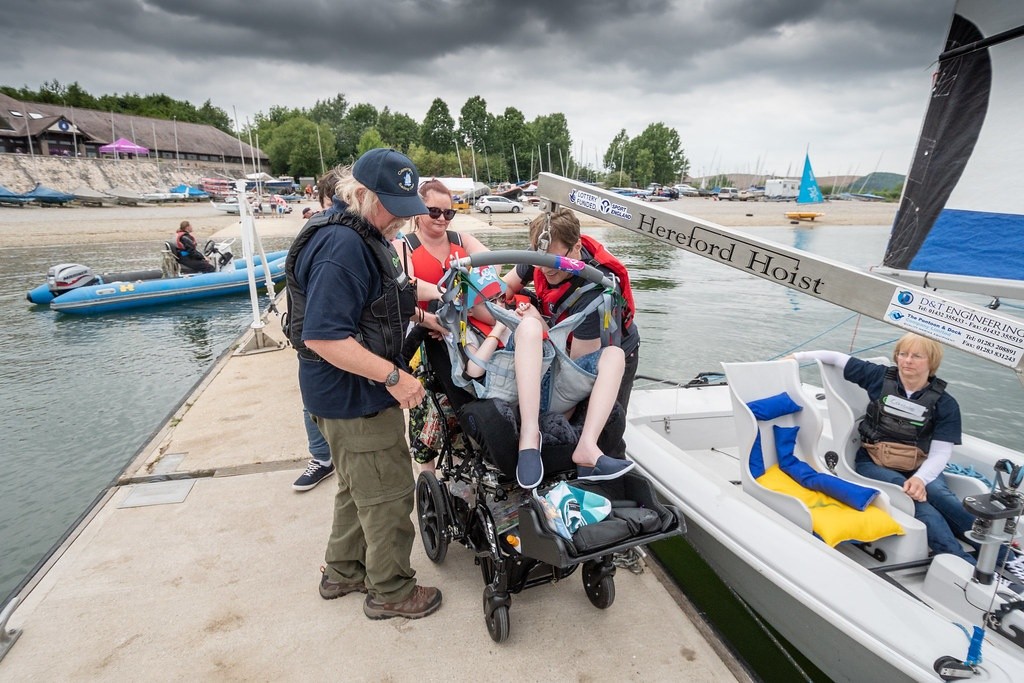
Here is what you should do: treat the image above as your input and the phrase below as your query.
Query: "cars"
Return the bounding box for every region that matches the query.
[475,195,524,214]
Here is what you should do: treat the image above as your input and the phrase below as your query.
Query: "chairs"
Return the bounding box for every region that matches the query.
[720,357,905,548]
[815,356,993,555]
[164,241,197,273]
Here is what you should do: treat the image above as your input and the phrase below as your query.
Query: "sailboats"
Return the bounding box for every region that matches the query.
[488,140,764,202]
[0,104,304,216]
[825,154,887,202]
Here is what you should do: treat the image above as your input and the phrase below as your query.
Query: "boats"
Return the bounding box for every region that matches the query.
[784,212,823,221]
[622,350,1024,682]
[24,247,291,314]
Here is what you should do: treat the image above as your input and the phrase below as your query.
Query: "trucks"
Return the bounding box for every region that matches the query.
[764,179,802,202]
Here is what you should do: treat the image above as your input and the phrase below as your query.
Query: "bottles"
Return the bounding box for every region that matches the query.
[506,534,521,554]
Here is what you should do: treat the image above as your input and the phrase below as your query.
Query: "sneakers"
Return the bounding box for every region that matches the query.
[577,455,635,480]
[364,585,442,620]
[319,566,368,600]
[294,460,335,491]
[516,431,544,488]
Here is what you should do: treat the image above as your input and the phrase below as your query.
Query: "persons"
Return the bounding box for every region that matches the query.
[290,147,442,619]
[499,210,636,461]
[305,183,312,197]
[780,332,1016,570]
[292,169,348,492]
[270,192,276,218]
[253,199,259,219]
[303,208,319,219]
[312,184,319,199]
[392,181,492,312]
[277,197,286,218]
[466,272,635,490]
[176,220,215,272]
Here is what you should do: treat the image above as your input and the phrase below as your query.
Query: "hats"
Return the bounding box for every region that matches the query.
[455,265,507,310]
[353,148,430,217]
[303,208,310,219]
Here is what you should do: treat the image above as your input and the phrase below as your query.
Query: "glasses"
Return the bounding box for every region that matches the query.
[532,249,571,268]
[490,292,507,304]
[427,207,457,221]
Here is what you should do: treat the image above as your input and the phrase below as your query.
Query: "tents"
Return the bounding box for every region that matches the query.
[100,138,150,157]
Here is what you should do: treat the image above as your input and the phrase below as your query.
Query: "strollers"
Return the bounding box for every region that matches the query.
[415,249,689,644]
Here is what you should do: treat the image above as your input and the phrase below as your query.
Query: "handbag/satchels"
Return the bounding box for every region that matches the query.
[419,393,459,451]
[865,442,928,471]
